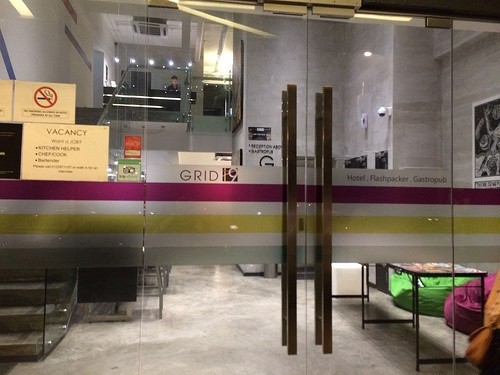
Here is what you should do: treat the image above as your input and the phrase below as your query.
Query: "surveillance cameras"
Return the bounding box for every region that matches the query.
[378,107,387,118]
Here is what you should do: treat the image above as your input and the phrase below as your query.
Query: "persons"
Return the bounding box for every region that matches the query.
[167,75,179,90]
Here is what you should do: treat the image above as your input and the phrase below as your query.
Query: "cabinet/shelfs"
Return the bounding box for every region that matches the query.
[1,268,79,363]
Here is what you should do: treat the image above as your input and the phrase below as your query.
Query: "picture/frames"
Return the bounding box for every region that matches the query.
[231,40,243,133]
[472,95,500,189]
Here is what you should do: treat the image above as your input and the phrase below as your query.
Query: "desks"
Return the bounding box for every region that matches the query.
[358,263,488,373]
